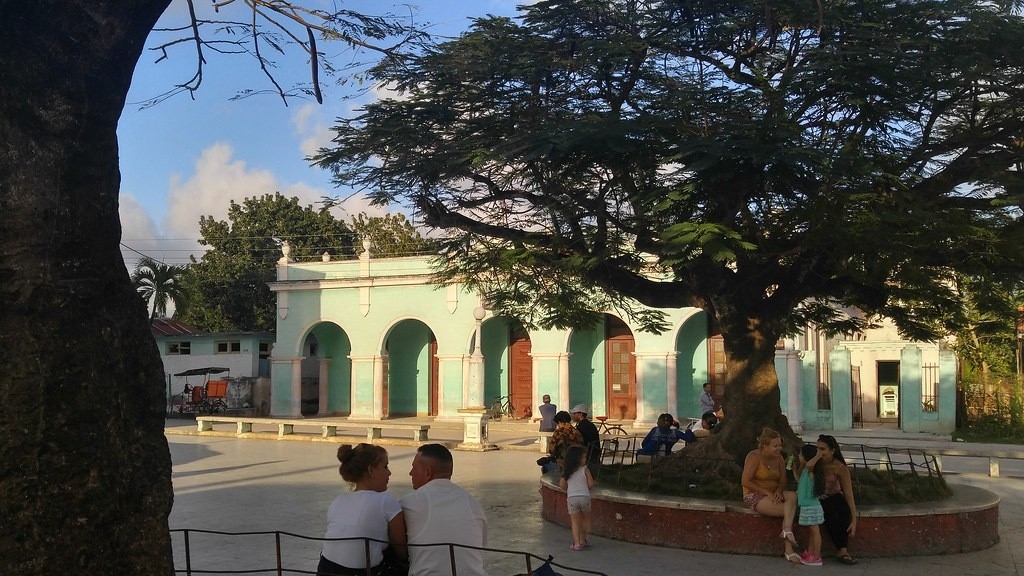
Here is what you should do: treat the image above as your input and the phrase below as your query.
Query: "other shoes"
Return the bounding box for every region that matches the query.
[537,457,552,465]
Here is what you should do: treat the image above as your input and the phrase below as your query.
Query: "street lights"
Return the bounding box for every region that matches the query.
[452,306,503,452]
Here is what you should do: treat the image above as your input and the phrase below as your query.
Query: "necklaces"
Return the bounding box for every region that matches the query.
[764,458,776,473]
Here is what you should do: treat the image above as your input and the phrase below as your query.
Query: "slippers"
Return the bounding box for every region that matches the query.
[838,553,857,565]
[569,543,582,550]
[579,539,592,547]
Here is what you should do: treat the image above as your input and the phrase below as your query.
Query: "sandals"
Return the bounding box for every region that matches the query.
[778,530,797,545]
[784,552,801,563]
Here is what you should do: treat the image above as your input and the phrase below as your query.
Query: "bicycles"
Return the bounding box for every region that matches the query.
[684,403,724,431]
[490,393,519,423]
[571,416,631,453]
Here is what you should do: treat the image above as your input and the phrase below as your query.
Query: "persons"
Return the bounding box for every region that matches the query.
[562,447,594,550]
[742,429,800,563]
[797,444,823,566]
[816,435,857,564]
[398,444,490,576]
[533,394,557,444]
[316,443,408,576]
[537,404,601,475]
[635,413,697,463]
[691,405,725,439]
[699,382,714,414]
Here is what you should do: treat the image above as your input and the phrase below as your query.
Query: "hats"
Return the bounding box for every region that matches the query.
[570,404,588,413]
[543,395,550,402]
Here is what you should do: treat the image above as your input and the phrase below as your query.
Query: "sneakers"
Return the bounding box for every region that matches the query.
[800,555,823,566]
[799,550,812,560]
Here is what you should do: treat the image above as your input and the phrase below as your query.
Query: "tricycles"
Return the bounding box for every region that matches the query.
[169,365,230,419]
